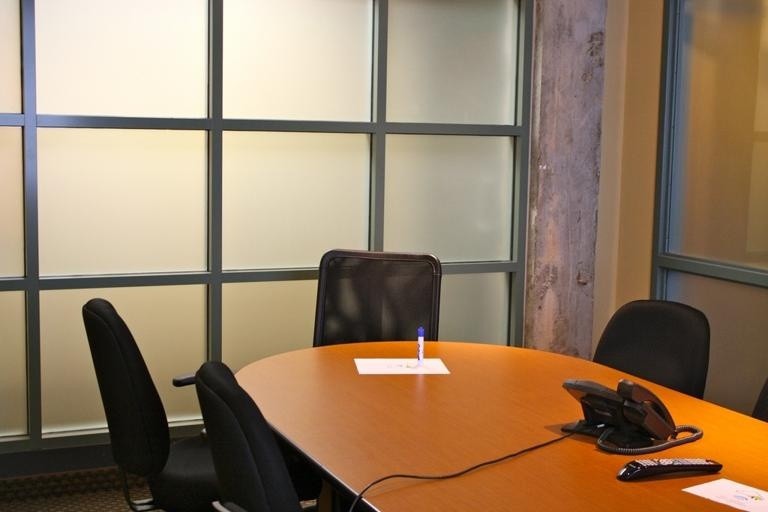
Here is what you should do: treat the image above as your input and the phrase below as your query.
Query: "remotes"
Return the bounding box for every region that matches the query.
[616,458,723,481]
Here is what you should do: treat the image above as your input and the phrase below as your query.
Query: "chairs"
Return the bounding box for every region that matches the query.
[193,361,366,512]
[81,297,322,512]
[313,250,443,348]
[592,299,710,400]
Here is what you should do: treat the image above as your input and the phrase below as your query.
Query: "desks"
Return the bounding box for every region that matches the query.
[228,339,767,512]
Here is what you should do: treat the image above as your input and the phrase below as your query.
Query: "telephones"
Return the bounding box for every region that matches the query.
[561,378,678,448]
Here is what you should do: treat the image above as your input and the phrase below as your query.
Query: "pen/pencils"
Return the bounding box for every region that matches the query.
[417,327,424,367]
